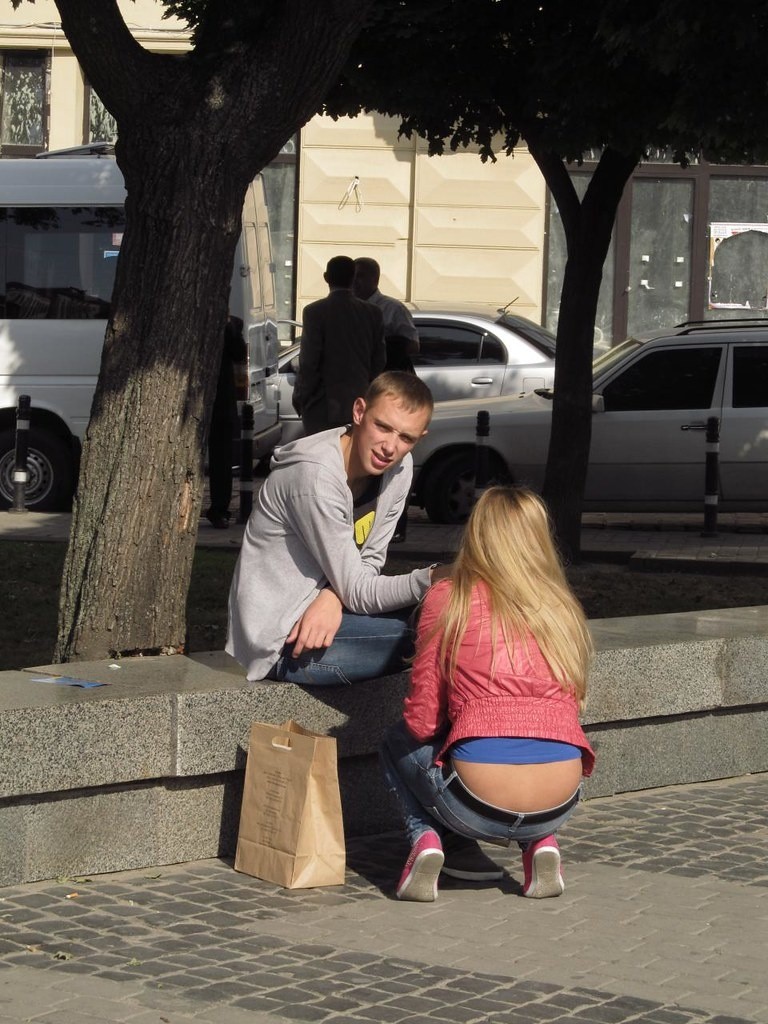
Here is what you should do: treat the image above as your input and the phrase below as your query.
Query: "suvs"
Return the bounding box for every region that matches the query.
[411,318,768,525]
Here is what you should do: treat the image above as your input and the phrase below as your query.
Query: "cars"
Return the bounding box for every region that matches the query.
[278,310,609,446]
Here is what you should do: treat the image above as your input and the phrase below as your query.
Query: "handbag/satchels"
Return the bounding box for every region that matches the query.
[233,720,346,889]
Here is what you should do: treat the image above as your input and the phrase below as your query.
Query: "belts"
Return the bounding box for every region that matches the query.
[440,751,580,825]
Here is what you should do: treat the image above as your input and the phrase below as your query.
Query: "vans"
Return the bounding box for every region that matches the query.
[1,143,282,511]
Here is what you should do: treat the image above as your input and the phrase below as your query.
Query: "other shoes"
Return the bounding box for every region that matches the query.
[425,812,505,880]
[206,509,229,528]
[396,830,445,901]
[388,527,406,543]
[235,514,249,523]
[522,833,565,898]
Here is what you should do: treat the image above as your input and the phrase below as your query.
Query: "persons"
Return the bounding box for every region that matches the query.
[291,255,388,436]
[65,244,115,301]
[223,369,506,881]
[206,314,246,528]
[349,256,420,541]
[375,483,595,904]
